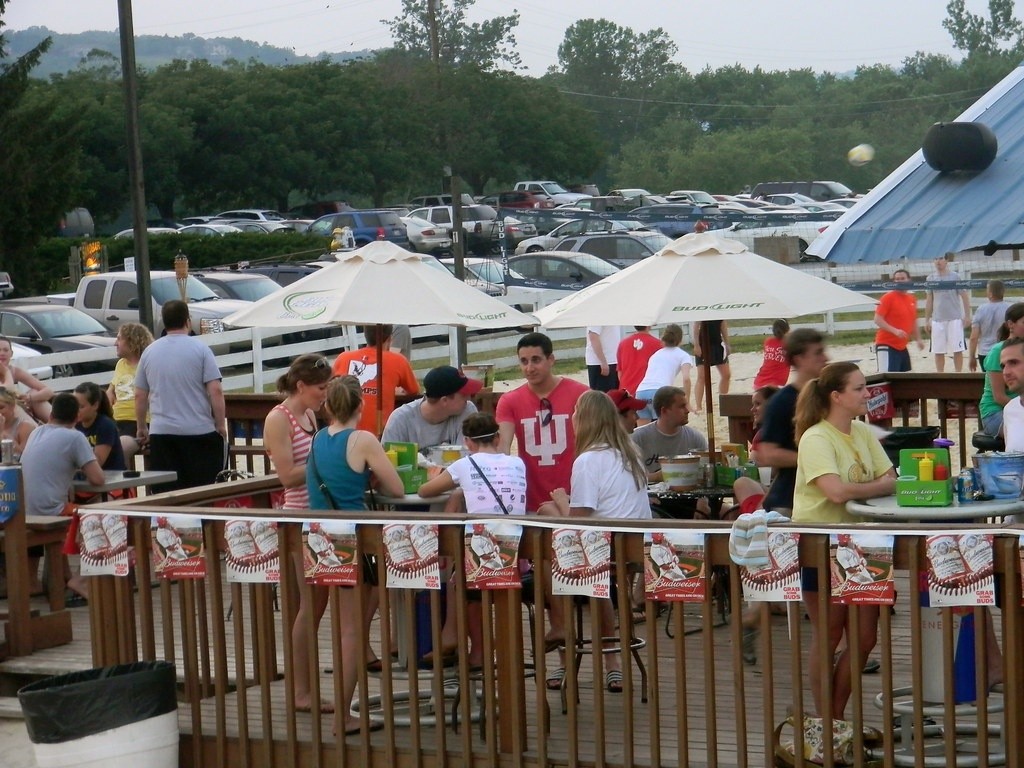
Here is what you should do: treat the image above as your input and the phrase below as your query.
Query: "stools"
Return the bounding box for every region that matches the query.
[972,432,1005,454]
[556,560,647,715]
[881,425,941,470]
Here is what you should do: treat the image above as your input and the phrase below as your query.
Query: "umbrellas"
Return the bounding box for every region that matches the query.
[532,222,883,514]
[804,59,1024,264]
[221,228,538,442]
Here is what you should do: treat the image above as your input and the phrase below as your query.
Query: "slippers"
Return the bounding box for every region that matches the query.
[546,664,565,689]
[295,696,334,714]
[605,669,623,692]
[415,653,459,670]
[333,720,383,736]
[529,638,565,657]
[366,660,382,674]
[66,592,89,608]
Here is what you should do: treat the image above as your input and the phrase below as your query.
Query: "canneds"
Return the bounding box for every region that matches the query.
[735,466,747,481]
[958,467,974,504]
[703,463,716,488]
[1,438,14,465]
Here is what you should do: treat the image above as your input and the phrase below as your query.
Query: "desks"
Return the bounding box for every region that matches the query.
[845,492,1024,768]
[349,487,501,726]
[645,485,735,638]
[72,469,177,502]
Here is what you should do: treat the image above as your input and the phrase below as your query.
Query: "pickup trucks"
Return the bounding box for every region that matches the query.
[474,181,593,209]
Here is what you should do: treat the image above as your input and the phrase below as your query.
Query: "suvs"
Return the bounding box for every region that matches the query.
[73,270,332,372]
[550,229,674,270]
[302,210,410,252]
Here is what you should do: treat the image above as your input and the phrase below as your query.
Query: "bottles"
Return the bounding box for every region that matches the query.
[919,451,933,481]
[932,461,949,480]
[386,445,398,468]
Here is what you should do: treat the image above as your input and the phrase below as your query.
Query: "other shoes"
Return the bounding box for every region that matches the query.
[863,658,882,672]
[742,625,758,663]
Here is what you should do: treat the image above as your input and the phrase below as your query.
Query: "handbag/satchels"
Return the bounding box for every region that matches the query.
[772,707,878,768]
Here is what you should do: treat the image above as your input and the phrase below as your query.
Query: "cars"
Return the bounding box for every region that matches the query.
[178,208,315,237]
[607,188,871,239]
[112,226,186,238]
[440,256,529,297]
[513,217,653,260]
[0,271,15,299]
[0,335,53,399]
[215,261,321,287]
[366,191,549,254]
[506,250,622,290]
[751,180,852,199]
[0,304,121,376]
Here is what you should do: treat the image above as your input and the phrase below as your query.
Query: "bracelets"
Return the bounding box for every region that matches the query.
[29,394,32,403]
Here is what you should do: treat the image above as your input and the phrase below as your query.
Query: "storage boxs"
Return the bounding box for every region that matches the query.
[398,466,427,493]
[715,443,759,487]
[896,477,954,507]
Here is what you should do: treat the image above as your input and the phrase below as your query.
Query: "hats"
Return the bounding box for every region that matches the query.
[423,366,484,397]
[606,389,646,410]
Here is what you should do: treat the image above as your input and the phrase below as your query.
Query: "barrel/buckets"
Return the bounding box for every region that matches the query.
[461,363,494,392]
[688,447,727,487]
[658,452,701,492]
[972,453,1024,499]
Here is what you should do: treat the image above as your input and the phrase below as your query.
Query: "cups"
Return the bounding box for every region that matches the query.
[896,475,917,481]
[757,467,771,486]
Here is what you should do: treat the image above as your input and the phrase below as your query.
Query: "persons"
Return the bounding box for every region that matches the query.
[969,280,1024,695]
[262,318,899,737]
[874,269,925,372]
[924,256,972,372]
[0,300,227,606]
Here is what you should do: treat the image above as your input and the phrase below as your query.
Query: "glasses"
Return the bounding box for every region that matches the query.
[309,356,329,371]
[539,397,553,426]
[617,388,630,406]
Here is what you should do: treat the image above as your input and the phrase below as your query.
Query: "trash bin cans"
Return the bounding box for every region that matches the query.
[881,426,942,469]
[16,660,179,768]
